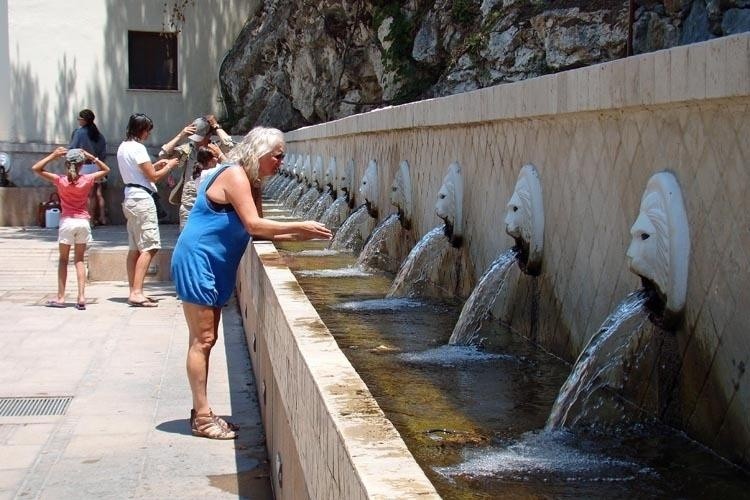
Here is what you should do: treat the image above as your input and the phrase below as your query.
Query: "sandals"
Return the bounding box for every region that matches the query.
[190,409,240,439]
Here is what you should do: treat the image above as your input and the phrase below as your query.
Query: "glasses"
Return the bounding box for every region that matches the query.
[76,117,85,121]
[271,155,286,161]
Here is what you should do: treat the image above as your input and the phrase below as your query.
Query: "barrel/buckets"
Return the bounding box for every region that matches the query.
[45,208,60,228]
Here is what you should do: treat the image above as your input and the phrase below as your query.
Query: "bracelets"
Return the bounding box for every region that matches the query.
[93,157,99,164]
[217,152,224,160]
[214,125,223,129]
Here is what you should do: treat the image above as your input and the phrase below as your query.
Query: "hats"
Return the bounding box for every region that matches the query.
[65,148,86,163]
[188,117,208,142]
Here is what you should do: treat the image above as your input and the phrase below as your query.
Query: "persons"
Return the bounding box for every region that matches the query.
[193,141,228,196]
[162,118,235,235]
[66,110,109,226]
[171,127,333,439]
[116,113,180,307]
[33,146,111,309]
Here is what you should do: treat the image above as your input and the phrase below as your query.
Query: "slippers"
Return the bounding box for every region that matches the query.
[76,302,86,310]
[47,299,64,308]
[127,297,158,306]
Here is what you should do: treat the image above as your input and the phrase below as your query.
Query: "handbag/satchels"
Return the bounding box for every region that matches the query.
[151,192,168,217]
[170,180,185,205]
[39,203,61,227]
